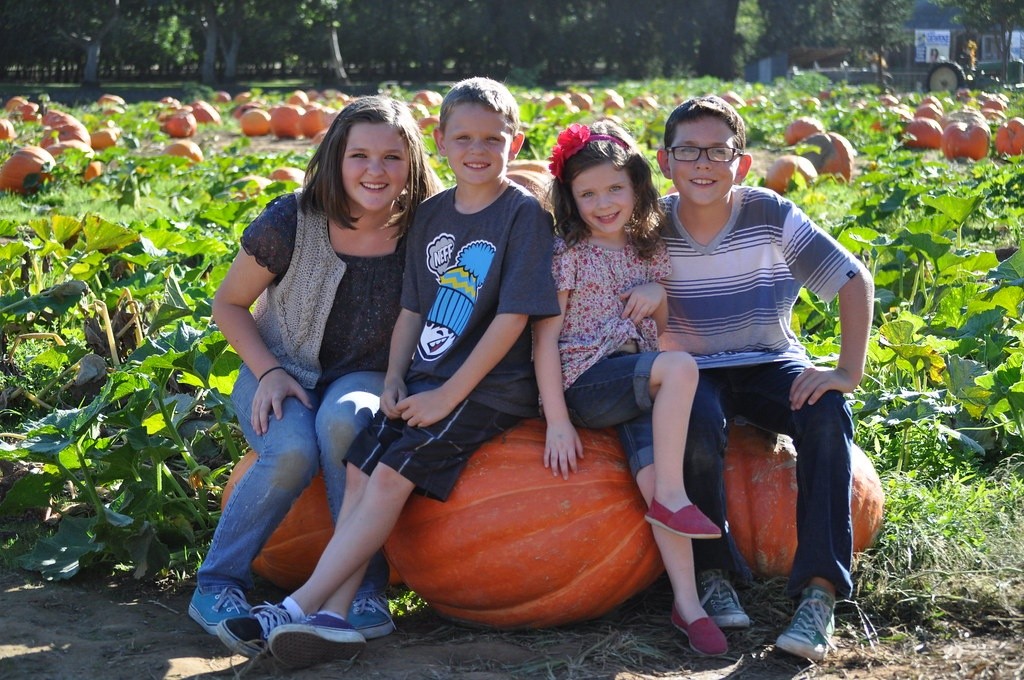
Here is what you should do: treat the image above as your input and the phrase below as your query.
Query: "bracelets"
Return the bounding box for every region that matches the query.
[256,366,287,384]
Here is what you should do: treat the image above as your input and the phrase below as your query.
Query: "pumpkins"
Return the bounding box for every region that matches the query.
[723,421,884,571]
[385,418,665,628]
[220,448,335,595]
[1,89,1024,192]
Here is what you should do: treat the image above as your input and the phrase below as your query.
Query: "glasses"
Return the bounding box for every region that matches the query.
[666,146,745,162]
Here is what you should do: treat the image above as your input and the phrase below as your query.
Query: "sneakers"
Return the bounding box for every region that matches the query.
[187,585,252,636]
[345,593,397,639]
[217,600,294,658]
[268,613,367,668]
[774,585,839,661]
[695,568,750,628]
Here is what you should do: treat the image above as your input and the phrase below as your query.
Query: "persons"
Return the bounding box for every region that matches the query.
[218,78,564,664]
[634,98,874,662]
[182,93,432,653]
[533,120,734,660]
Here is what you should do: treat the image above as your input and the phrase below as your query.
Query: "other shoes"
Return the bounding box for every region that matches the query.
[671,600,728,657]
[643,495,721,539]
